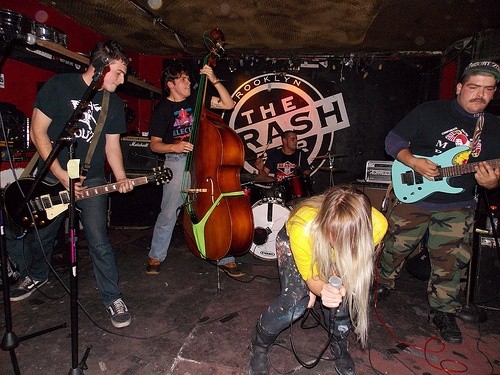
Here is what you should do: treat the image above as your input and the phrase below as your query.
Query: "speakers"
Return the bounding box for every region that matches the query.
[471,232,500,311]
[353,182,397,217]
[107,172,163,230]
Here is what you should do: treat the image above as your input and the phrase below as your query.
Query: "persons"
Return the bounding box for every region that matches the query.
[8,41,135,329]
[256,130,312,202]
[369,60,500,343]
[247,183,388,375]
[147,64,244,278]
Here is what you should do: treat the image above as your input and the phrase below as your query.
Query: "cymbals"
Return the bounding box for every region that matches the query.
[311,155,346,159]
[241,173,277,183]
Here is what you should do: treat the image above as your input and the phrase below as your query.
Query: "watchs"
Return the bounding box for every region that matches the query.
[212,78,220,85]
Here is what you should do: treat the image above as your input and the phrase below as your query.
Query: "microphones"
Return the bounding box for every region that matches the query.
[328,276,343,328]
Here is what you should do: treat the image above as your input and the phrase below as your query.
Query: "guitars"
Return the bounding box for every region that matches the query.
[391,144,500,204]
[3,165,173,234]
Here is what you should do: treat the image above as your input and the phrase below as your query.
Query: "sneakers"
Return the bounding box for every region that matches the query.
[10,275,48,302]
[220,262,245,277]
[146,258,161,275]
[107,298,131,328]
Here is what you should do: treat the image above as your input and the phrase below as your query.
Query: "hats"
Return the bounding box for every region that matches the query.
[461,61,500,82]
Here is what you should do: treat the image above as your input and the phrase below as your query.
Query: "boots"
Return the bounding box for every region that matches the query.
[250,314,277,375]
[331,316,355,375]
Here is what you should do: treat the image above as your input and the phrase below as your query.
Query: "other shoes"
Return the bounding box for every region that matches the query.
[370,284,389,308]
[432,311,461,342]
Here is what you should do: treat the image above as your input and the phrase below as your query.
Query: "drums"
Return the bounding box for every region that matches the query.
[286,174,313,200]
[249,197,292,262]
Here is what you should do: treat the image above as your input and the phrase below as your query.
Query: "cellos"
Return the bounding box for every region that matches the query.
[181,27,255,289]
